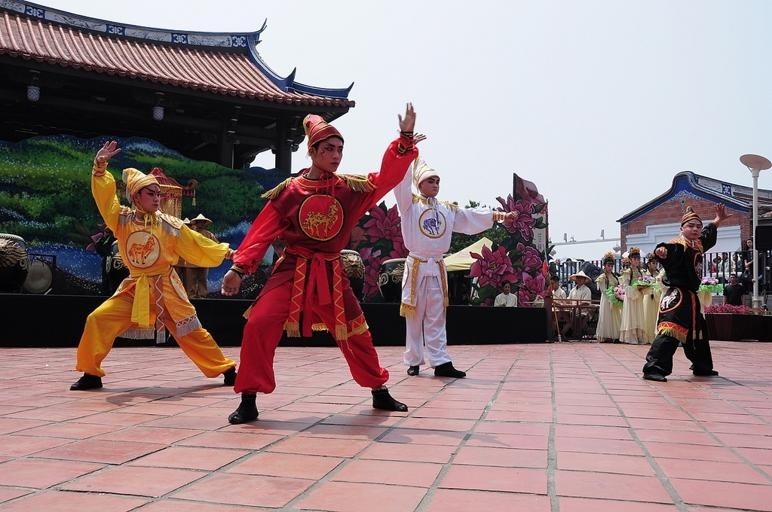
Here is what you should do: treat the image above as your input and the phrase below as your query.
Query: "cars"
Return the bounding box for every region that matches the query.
[552,262,601,295]
[710,267,745,284]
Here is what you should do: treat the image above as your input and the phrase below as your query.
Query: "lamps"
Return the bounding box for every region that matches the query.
[26,67,45,105]
[151,90,166,120]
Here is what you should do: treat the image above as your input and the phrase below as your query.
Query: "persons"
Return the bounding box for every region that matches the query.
[639,202,735,384]
[221,96,417,426]
[185,208,220,300]
[494,278,518,309]
[67,137,239,388]
[536,234,771,347]
[392,132,520,380]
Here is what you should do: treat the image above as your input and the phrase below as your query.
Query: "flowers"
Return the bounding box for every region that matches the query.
[351,197,415,302]
[632,273,661,295]
[696,274,725,294]
[468,184,557,307]
[607,281,627,310]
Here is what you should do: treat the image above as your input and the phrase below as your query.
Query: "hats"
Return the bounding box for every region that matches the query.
[569,271,592,285]
[302,113,345,151]
[413,159,441,188]
[681,206,703,228]
[190,214,213,225]
[183,218,191,224]
[122,168,160,205]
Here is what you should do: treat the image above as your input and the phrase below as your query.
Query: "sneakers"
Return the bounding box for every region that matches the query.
[693,370,719,376]
[643,373,668,382]
[435,362,466,378]
[70,372,103,390]
[229,393,259,423]
[371,388,407,412]
[408,366,419,376]
[223,368,237,386]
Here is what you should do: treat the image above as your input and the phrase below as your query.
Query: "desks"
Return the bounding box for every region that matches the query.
[548,296,606,341]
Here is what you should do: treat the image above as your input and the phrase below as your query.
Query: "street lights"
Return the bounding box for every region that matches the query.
[736,152,772,312]
